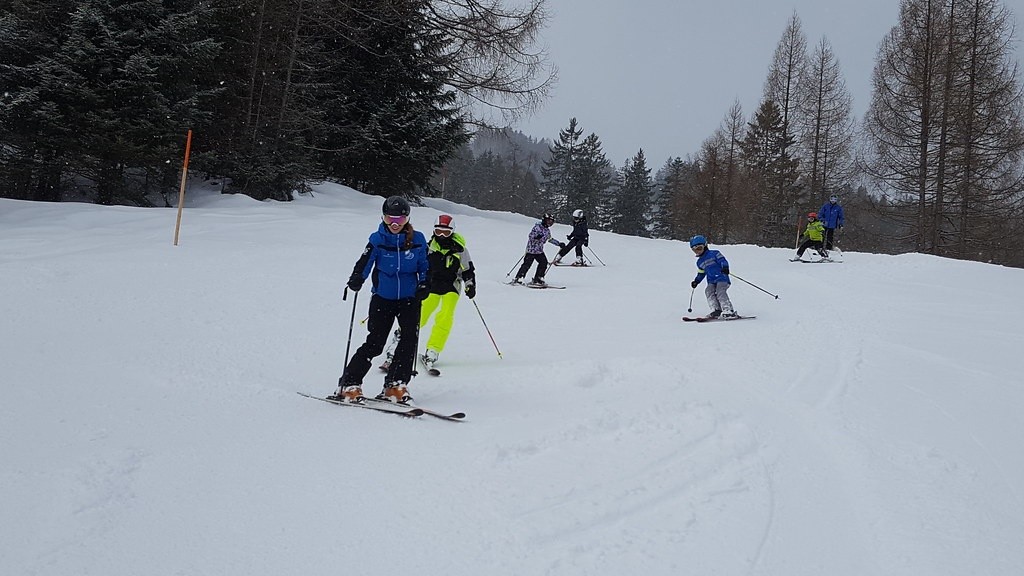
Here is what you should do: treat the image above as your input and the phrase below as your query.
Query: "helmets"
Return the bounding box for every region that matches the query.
[434,215,455,232]
[541,213,554,226]
[383,196,410,217]
[830,194,838,201]
[808,212,818,219]
[573,209,584,220]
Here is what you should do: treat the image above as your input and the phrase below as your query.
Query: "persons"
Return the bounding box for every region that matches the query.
[384,215,476,367]
[556,209,589,265]
[514,213,565,285]
[794,213,830,262]
[690,235,737,318]
[336,197,429,403]
[819,195,844,250]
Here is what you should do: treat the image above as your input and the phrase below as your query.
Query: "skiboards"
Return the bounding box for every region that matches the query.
[682,315,757,323]
[295,390,467,419]
[813,253,817,255]
[548,262,595,267]
[378,353,441,376]
[498,280,566,289]
[789,258,844,264]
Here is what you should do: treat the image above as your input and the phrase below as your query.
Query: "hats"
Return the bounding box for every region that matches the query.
[690,235,706,248]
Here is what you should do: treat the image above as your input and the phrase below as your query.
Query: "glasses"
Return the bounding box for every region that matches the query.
[692,245,704,252]
[383,215,408,226]
[434,227,453,237]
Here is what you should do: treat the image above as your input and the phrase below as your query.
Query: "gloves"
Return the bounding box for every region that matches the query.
[536,234,542,239]
[567,235,572,239]
[691,281,697,288]
[465,280,476,299]
[584,242,588,247]
[348,273,363,291]
[559,243,565,248]
[721,268,729,274]
[799,234,804,238]
[415,282,430,300]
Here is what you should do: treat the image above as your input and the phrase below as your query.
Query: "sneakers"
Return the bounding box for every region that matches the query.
[723,310,735,318]
[576,256,582,263]
[425,349,438,361]
[336,385,364,401]
[384,385,410,403]
[386,341,398,356]
[514,279,522,284]
[533,277,545,284]
[710,310,721,317]
